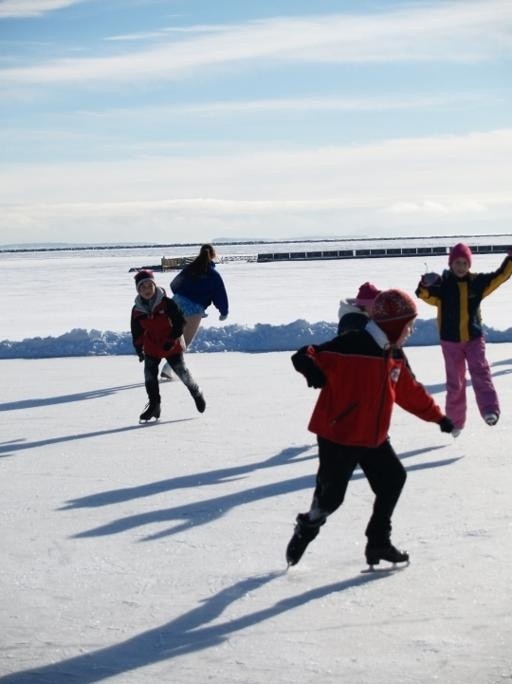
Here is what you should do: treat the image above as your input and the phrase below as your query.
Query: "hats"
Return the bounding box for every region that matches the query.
[449,243,472,266]
[372,290,416,343]
[135,271,154,291]
[356,282,378,303]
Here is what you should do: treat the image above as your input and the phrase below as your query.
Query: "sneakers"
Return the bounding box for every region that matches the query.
[485,412,497,425]
[161,368,173,379]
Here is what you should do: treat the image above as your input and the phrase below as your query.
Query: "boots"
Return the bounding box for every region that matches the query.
[366,517,408,564]
[194,391,205,412]
[140,395,160,419]
[286,513,326,563]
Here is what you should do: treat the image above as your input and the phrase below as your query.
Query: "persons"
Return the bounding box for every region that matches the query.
[336,279,383,335]
[285,288,457,569]
[130,268,206,420]
[161,243,231,381]
[414,242,512,435]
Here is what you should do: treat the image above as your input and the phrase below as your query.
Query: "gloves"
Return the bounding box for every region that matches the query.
[136,349,144,361]
[421,273,442,287]
[165,337,174,350]
[302,366,325,388]
[220,315,225,320]
[440,416,453,432]
